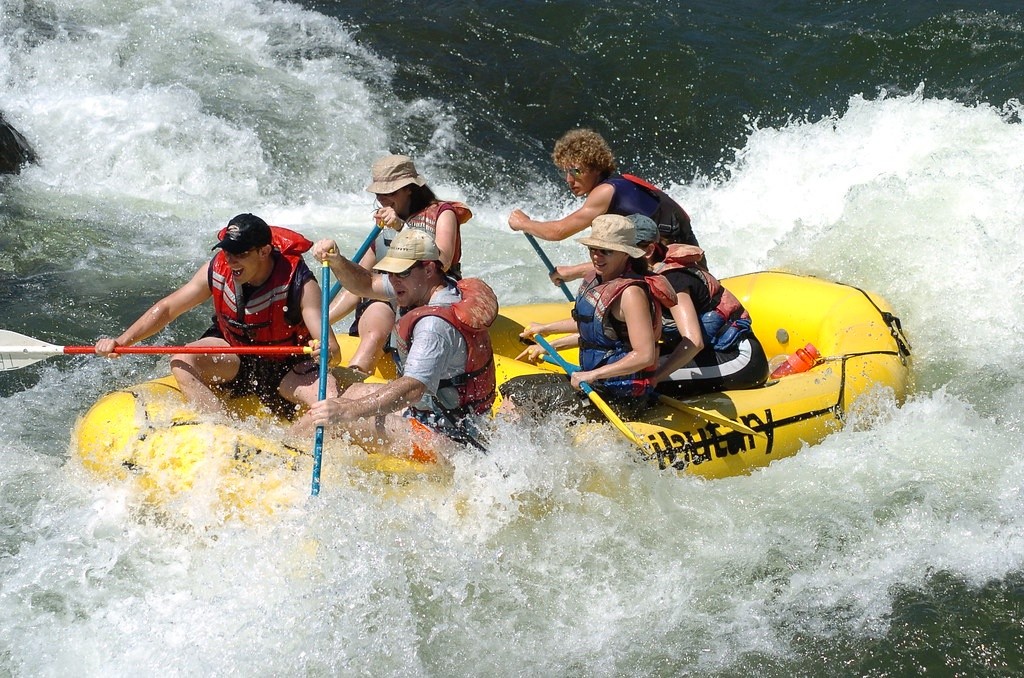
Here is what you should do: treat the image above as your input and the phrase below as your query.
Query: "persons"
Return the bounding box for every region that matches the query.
[498,214,678,427]
[508,129,708,287]
[308,229,496,464]
[93,213,341,457]
[327,154,462,374]
[515,214,769,399]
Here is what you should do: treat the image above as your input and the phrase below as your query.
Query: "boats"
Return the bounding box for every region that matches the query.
[73,270,912,528]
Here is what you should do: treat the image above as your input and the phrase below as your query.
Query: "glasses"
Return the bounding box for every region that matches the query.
[225,245,264,260]
[380,264,424,277]
[588,246,628,256]
[556,167,595,179]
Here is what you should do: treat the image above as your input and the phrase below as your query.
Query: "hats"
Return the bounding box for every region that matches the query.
[211,212,272,256]
[372,227,439,273]
[574,214,646,259]
[626,213,660,245]
[366,156,426,194]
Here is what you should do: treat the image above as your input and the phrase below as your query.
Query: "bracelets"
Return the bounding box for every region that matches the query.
[397,223,404,232]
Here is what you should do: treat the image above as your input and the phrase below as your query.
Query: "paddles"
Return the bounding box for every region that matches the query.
[528,348,769,438]
[327,220,384,306]
[523,229,574,302]
[310,246,337,496]
[525,325,655,461]
[1,328,308,373]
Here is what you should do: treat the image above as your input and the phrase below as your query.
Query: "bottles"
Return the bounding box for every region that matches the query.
[769,343,823,378]
